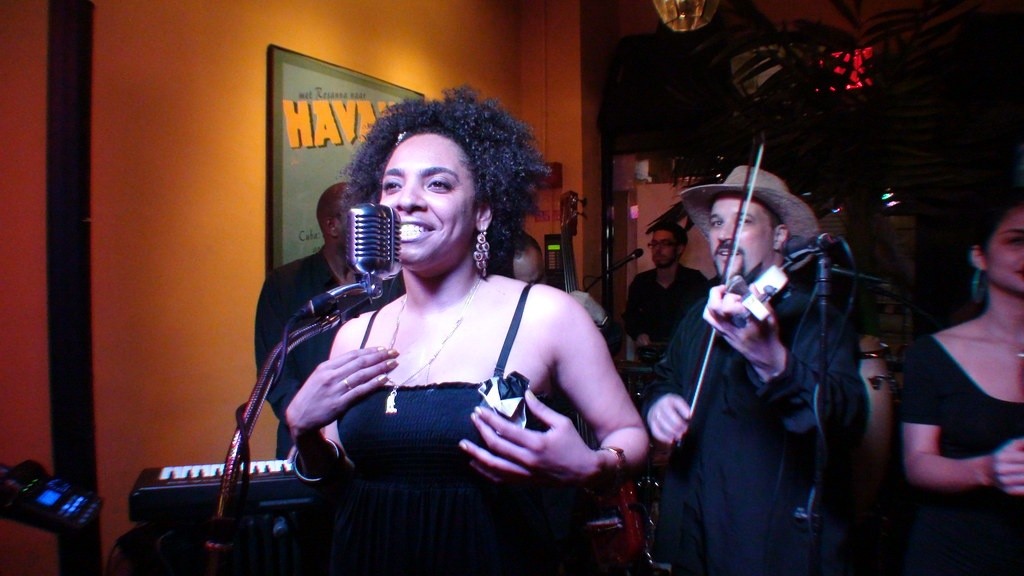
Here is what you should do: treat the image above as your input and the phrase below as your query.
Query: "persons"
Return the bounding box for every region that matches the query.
[624,221,710,364]
[785,250,892,505]
[255,181,396,461]
[285,85,650,576]
[511,229,548,285]
[902,201,1024,576]
[646,166,870,576]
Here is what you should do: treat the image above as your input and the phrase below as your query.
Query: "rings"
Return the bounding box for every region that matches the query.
[655,414,660,420]
[344,379,351,391]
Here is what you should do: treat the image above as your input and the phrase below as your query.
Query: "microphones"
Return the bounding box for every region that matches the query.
[782,233,841,266]
[292,204,402,321]
[611,247,644,271]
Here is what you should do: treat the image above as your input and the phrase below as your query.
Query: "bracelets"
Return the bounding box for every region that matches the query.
[601,446,626,471]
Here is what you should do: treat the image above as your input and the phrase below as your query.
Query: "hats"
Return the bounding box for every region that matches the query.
[681,165,819,249]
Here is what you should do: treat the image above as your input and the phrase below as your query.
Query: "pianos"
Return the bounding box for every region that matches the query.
[127,457,340,525]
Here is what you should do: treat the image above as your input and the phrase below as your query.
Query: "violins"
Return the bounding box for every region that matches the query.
[722,264,790,322]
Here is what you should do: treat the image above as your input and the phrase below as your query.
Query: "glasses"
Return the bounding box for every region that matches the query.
[647,239,674,250]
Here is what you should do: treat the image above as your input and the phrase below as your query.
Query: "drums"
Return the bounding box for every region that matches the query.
[845,332,898,525]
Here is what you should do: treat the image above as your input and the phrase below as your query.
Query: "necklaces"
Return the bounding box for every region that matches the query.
[386,278,482,414]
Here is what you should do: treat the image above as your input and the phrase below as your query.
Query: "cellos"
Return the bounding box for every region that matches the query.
[556,188,653,574]
